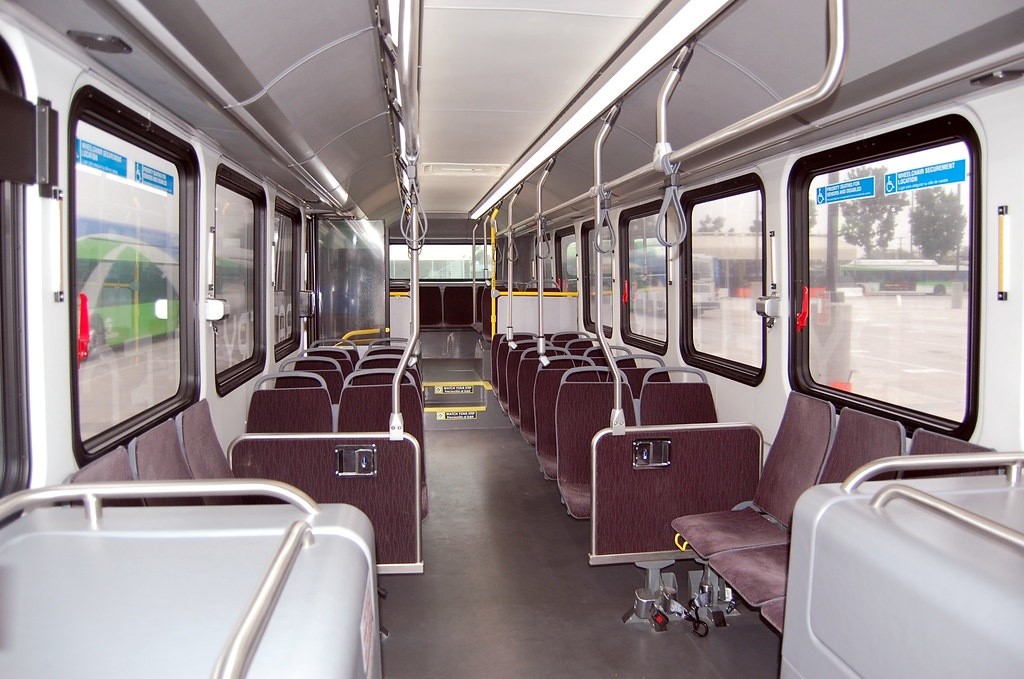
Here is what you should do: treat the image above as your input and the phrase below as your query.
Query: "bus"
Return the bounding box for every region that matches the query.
[632,253,720,317]
[837,257,968,297]
[219,255,253,313]
[76,233,180,351]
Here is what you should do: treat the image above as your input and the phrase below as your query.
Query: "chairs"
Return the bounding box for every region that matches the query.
[388,281,507,330]
[61,445,143,506]
[497,332,538,412]
[481,281,522,342]
[760,430,1001,635]
[362,346,424,381]
[550,332,590,348]
[294,347,355,378]
[555,366,639,520]
[608,355,671,399]
[491,333,534,398]
[533,355,602,481]
[128,418,205,506]
[518,346,577,448]
[638,366,719,426]
[275,356,346,404]
[581,345,637,367]
[350,355,425,408]
[308,338,360,370]
[564,338,612,356]
[181,398,255,505]
[524,279,562,341]
[368,337,422,365]
[506,339,559,426]
[337,369,429,518]
[708,406,907,607]
[246,371,334,435]
[671,389,837,559]
